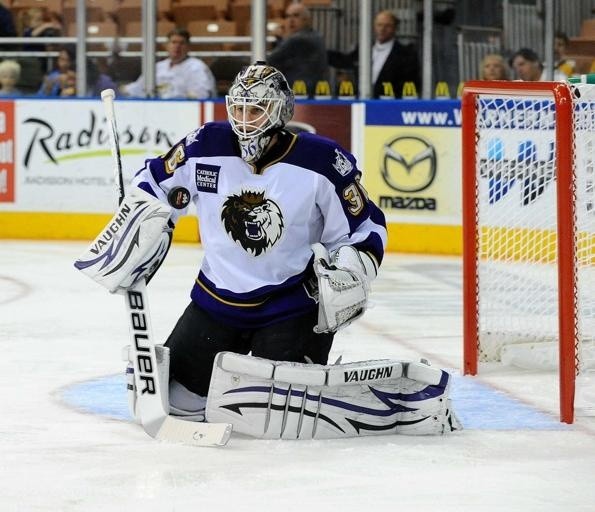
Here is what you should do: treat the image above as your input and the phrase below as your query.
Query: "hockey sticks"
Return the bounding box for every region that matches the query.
[100,88,233,446]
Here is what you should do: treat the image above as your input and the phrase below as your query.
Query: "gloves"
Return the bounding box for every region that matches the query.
[314,245,379,335]
[73,195,173,291]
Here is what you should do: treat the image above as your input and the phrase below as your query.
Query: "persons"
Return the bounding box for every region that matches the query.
[37,46,76,97]
[23,7,63,51]
[65,60,465,450]
[326,9,417,99]
[265,1,331,96]
[58,59,119,99]
[214,72,235,98]
[1,59,27,99]
[0,1,18,50]
[540,30,577,75]
[507,47,569,82]
[477,52,512,82]
[121,28,218,101]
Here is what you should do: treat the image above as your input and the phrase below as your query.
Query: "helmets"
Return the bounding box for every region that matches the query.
[224,64,294,164]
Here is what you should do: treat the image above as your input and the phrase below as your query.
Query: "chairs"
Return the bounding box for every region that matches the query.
[0,0,293,72]
[569,18,595,57]
